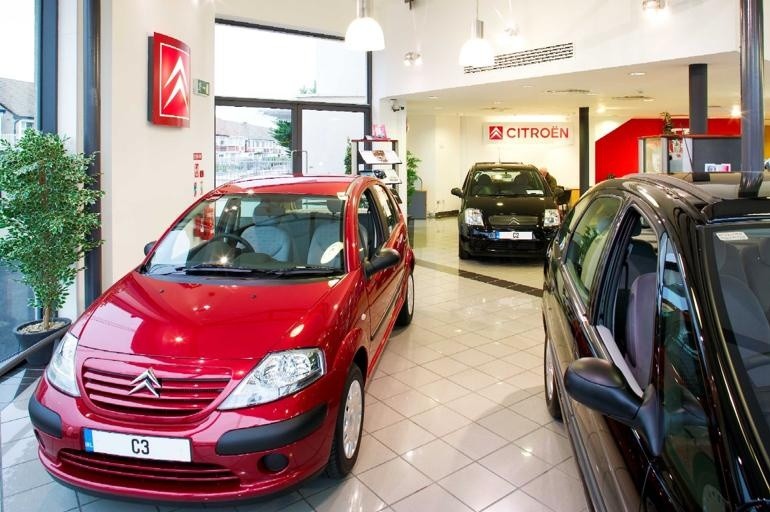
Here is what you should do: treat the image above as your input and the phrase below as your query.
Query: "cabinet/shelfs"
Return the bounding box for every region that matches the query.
[351,139,402,195]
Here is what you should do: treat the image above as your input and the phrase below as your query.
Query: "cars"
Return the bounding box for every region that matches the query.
[28,176,416,505]
[542,172,765,512]
[452,164,565,265]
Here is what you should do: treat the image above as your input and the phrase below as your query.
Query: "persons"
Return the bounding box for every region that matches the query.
[540,167,557,192]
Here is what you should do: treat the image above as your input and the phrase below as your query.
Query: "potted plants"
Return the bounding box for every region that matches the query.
[407,150,422,224]
[0,124,106,367]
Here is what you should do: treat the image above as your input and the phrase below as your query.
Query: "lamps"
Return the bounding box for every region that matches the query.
[459,1,495,67]
[345,0,386,52]
[642,0,665,12]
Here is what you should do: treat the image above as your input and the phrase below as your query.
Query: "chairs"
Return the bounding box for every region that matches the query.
[235,199,369,270]
[472,174,533,196]
[617,229,770,425]
